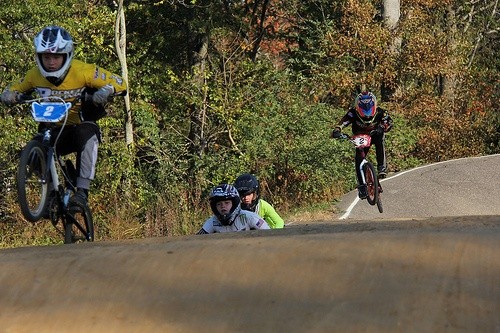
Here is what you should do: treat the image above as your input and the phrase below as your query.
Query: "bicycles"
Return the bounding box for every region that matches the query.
[340,130,384,213]
[7,89,128,244]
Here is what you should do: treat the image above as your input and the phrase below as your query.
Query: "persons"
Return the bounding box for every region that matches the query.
[0,27,127,213]
[195,184,272,235]
[332,91,394,199]
[233,173,285,229]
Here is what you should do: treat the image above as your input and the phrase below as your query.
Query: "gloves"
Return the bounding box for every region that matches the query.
[332,126,341,138]
[0,91,16,107]
[376,124,385,134]
[92,86,112,108]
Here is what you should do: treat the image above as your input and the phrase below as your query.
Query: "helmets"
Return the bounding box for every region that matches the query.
[209,184,242,226]
[34,26,74,87]
[354,91,378,123]
[234,174,260,212]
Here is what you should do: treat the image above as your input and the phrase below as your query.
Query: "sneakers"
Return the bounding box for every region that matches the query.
[70,191,87,212]
[355,183,368,199]
[377,165,388,175]
